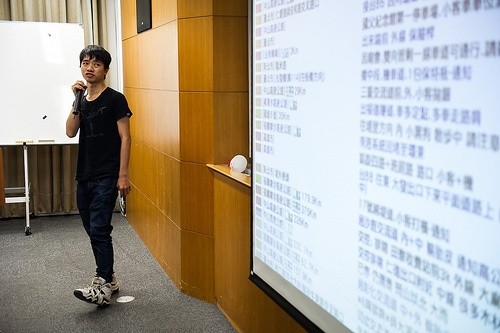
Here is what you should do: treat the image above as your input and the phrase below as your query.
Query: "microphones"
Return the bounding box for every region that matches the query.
[72,81,84,118]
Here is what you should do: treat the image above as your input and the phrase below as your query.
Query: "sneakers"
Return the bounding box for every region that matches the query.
[111,273,120,292]
[74,276,114,307]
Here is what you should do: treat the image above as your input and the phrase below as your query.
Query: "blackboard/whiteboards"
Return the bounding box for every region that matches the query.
[0,19,90,147]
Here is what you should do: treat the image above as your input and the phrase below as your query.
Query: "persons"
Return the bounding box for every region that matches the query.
[65,45,131,306]
